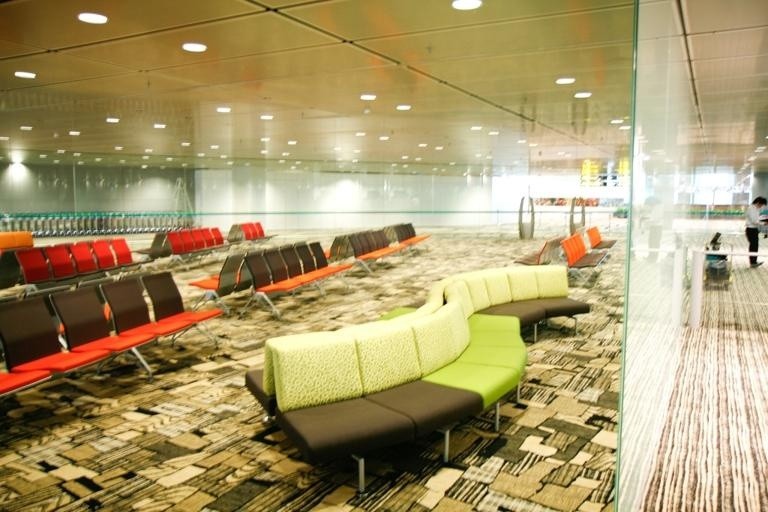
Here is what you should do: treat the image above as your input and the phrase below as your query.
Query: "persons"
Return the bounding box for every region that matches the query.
[744,196,768,268]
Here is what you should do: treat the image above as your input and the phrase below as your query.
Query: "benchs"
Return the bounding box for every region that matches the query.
[246,266,590,491]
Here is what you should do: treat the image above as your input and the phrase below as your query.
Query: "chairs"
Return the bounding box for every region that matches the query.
[513,227,616,286]
[2,219,225,400]
[327,220,432,278]
[133,219,354,325]
